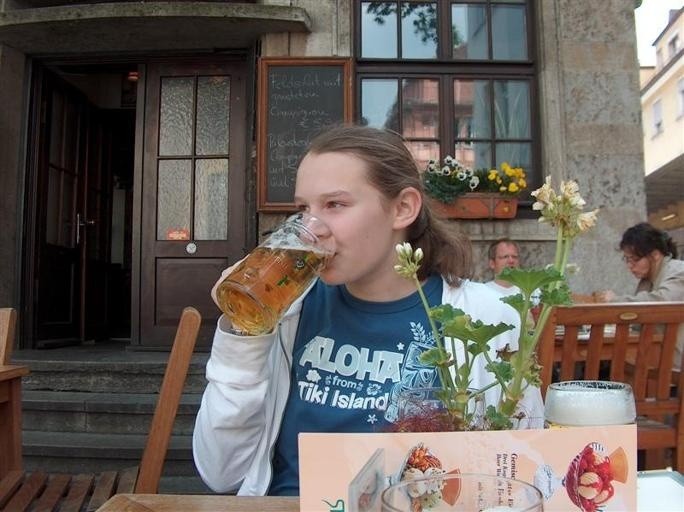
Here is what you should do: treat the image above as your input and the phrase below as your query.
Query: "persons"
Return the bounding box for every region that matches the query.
[482,238,542,330]
[600,222,683,385]
[194,126,542,498]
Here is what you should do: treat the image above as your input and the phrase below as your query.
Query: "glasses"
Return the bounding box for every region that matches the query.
[621,253,642,262]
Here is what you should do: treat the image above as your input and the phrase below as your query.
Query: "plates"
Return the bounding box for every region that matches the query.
[347,448,385,512]
[542,321,633,335]
[398,440,447,511]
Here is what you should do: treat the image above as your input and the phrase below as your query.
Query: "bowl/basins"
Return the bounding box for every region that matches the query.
[565,440,609,512]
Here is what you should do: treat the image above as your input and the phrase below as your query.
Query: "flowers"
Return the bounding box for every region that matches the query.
[422,155,480,205]
[393,175,599,430]
[490,161,526,196]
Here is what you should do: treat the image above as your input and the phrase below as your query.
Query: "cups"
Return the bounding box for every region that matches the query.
[396,387,486,430]
[383,472,547,512]
[215,212,336,334]
[545,381,636,425]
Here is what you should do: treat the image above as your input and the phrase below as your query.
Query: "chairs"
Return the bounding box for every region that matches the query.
[538,302,684,476]
[1,306,17,363]
[0,306,202,511]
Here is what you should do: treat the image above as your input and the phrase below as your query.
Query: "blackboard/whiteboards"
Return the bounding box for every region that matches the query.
[256,56,356,214]
